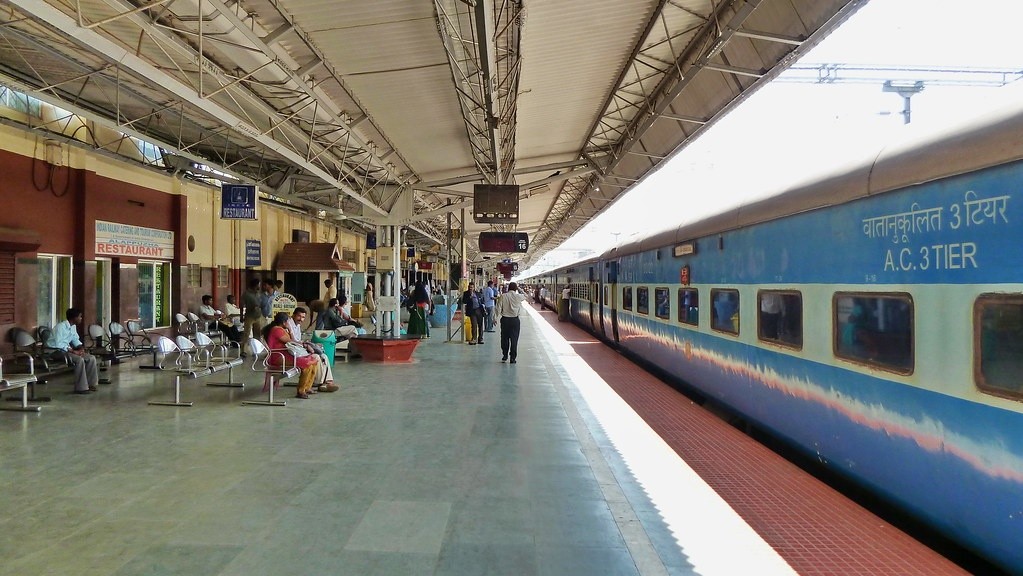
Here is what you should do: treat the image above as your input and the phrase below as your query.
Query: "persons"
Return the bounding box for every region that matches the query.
[305,299,328,330]
[199,294,243,349]
[42,306,99,395]
[238,278,266,358]
[273,280,283,292]
[259,278,281,342]
[492,281,529,363]
[260,312,319,400]
[224,295,245,341]
[324,278,335,308]
[337,296,367,335]
[284,307,340,392]
[324,299,359,338]
[366,275,573,346]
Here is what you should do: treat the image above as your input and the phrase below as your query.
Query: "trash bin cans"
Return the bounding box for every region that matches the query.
[311,330,337,369]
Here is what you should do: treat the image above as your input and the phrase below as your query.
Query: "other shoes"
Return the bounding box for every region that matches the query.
[298,383,339,398]
[471,330,519,365]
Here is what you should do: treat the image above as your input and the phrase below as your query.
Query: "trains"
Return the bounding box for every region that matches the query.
[517,79,1022,575]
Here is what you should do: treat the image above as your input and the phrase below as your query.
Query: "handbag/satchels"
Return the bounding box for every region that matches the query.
[286,341,310,357]
[304,340,324,355]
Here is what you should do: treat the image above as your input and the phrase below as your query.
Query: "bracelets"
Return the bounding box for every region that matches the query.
[288,331,293,335]
[72,349,75,353]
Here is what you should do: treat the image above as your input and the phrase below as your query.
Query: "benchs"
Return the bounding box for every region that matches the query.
[2,311,355,413]
